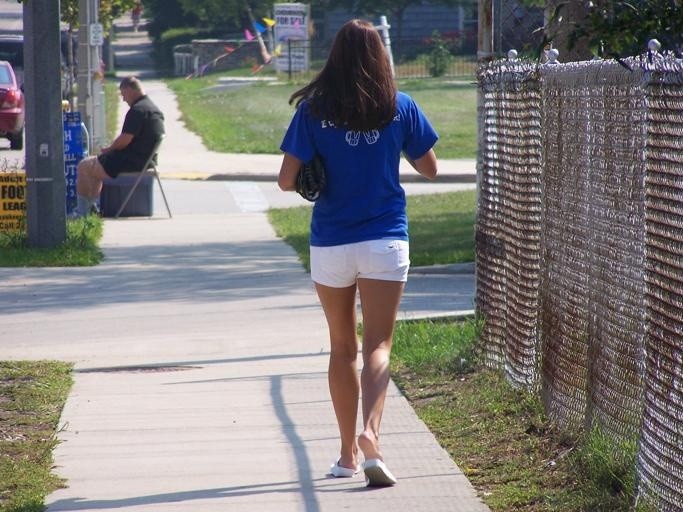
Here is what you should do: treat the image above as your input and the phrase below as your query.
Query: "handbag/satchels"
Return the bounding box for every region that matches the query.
[305,155,327,193]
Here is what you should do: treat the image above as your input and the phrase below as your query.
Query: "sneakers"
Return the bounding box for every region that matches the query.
[68,203,102,220]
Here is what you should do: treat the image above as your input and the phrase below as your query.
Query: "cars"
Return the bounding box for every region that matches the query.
[0,60,25,150]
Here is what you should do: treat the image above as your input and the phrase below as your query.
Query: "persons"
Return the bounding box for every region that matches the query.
[276,18,439,490]
[63,75,165,218]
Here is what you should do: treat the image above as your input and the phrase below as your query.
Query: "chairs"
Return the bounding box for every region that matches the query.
[103,133,173,218]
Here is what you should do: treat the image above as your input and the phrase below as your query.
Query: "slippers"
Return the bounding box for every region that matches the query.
[363,458,397,488]
[330,456,360,477]
